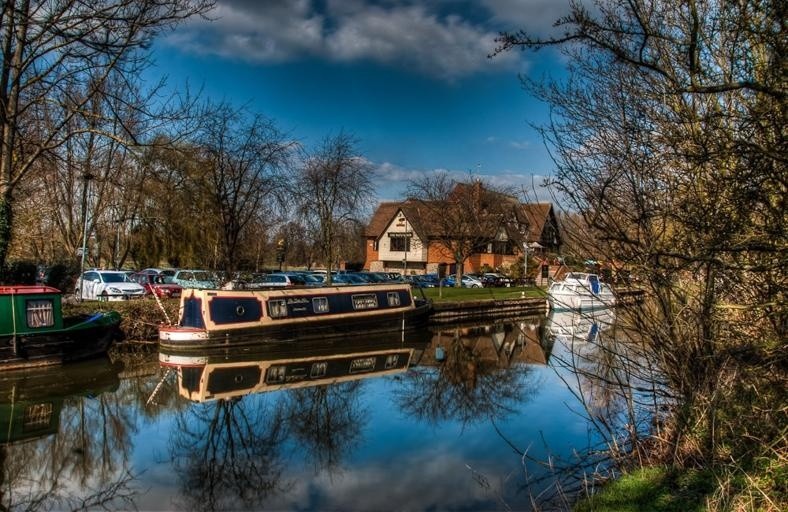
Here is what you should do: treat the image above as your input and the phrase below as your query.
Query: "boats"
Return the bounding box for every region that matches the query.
[545,271,618,311]
[157,334,429,406]
[157,283,430,347]
[548,310,616,343]
[1,286,124,364]
[0,350,124,448]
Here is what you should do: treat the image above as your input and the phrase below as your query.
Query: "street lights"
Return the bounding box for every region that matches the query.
[76,169,94,303]
